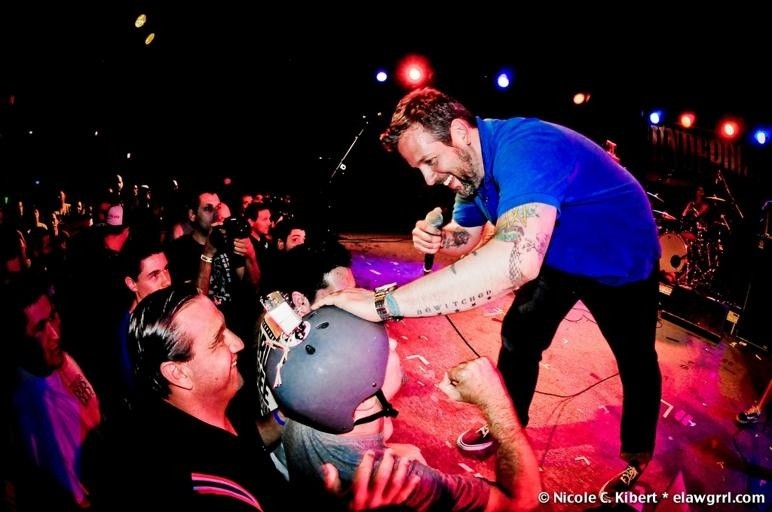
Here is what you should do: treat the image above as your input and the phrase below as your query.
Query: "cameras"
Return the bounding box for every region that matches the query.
[222,214,251,268]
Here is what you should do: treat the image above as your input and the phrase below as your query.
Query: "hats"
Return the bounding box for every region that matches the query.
[106,205,130,226]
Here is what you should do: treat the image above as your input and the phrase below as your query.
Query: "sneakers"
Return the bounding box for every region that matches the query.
[457,422,497,453]
[598,465,643,503]
[736,406,761,425]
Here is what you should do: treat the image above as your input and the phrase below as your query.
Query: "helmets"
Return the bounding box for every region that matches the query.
[264,306,389,435]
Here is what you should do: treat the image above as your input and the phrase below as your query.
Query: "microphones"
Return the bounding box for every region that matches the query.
[714,169,721,185]
[423,210,444,274]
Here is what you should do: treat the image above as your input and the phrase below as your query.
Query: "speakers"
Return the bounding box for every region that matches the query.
[661,286,730,344]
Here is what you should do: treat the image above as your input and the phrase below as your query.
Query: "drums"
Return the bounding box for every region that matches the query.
[658,231,704,280]
[709,220,731,250]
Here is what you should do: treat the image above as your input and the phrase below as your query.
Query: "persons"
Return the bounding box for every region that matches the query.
[735,379,772,424]
[682,184,710,218]
[0,170,546,511]
[311,87,663,501]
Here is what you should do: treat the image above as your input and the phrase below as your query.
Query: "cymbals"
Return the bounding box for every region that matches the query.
[648,192,663,201]
[654,210,677,221]
[705,196,725,201]
